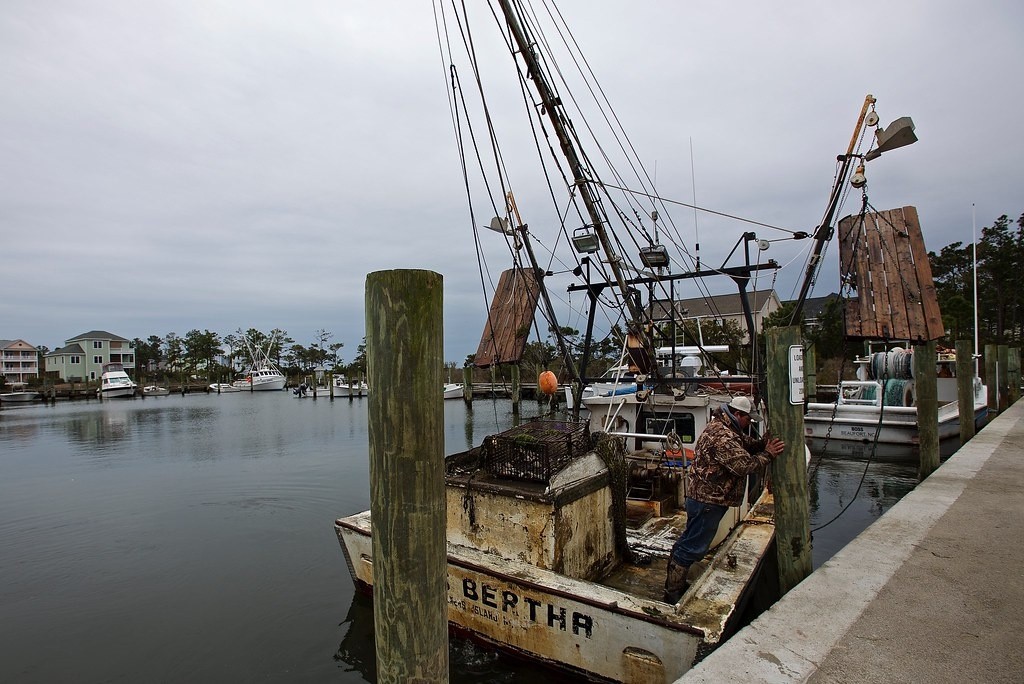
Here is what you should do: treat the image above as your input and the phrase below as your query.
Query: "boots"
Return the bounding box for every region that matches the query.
[664,555,689,604]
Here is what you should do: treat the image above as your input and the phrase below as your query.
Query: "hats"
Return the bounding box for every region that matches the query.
[729,395,764,423]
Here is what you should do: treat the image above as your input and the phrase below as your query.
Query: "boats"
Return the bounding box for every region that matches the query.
[0,392,38,401]
[144,386,170,395]
[301,374,367,396]
[444,382,463,398]
[209,383,240,393]
[96,362,138,398]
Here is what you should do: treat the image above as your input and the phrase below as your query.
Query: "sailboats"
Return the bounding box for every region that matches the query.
[332,1,809,684]
[233,327,286,390]
[803,203,988,444]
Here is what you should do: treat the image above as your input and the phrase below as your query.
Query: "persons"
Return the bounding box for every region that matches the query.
[662,391,788,605]
